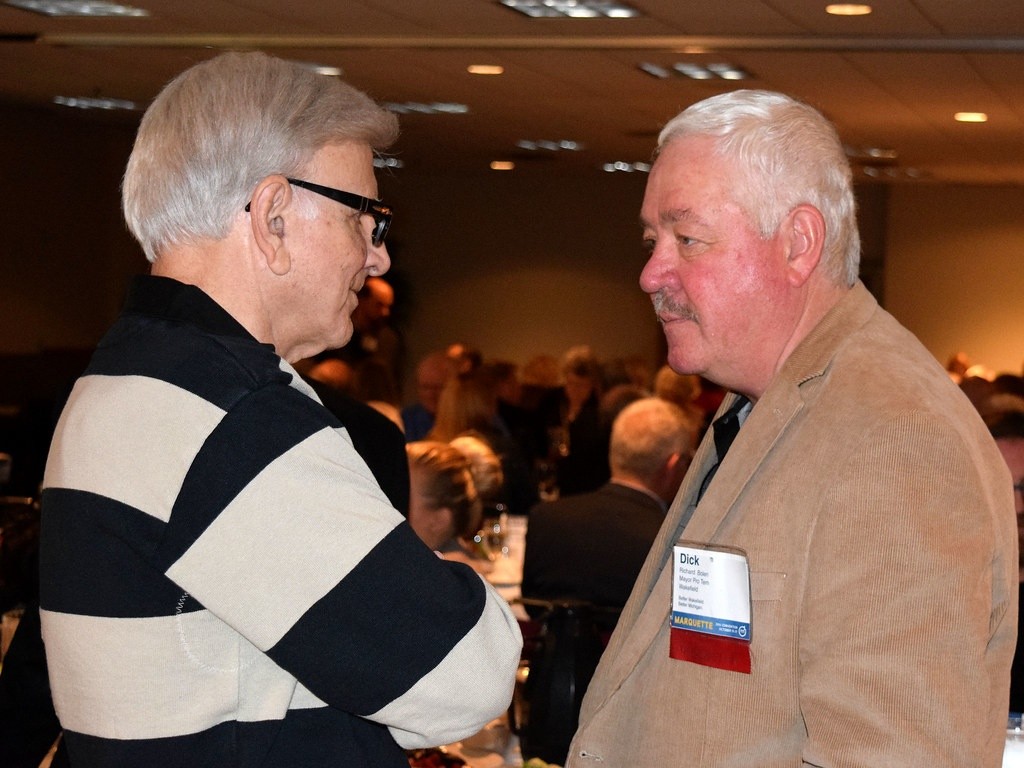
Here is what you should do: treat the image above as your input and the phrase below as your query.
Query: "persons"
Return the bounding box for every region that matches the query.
[0,48,693,768]
[569,89,1024,768]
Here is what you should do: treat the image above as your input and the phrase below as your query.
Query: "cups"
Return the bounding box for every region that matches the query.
[485,519,505,556]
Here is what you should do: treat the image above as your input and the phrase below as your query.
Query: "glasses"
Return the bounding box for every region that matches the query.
[245,177,392,248]
